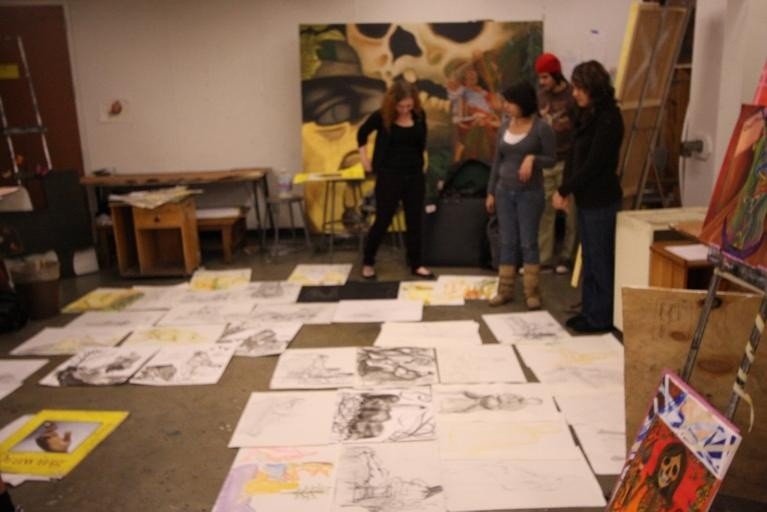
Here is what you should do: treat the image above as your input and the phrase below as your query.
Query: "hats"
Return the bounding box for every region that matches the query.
[534,52,561,74]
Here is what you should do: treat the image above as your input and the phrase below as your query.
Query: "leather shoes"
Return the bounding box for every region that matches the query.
[411,268,435,280]
[573,322,611,335]
[360,266,378,281]
[565,318,581,329]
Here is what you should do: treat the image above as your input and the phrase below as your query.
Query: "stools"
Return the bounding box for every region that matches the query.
[362,205,407,268]
[262,191,312,257]
[320,179,365,258]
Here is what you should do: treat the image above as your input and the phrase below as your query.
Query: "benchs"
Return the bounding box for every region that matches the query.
[96,205,251,269]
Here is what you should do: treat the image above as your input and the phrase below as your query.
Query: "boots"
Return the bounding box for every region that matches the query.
[523,263,543,309]
[488,265,517,307]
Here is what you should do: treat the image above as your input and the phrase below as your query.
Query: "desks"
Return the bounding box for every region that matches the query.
[79,166,275,252]
[611,207,716,346]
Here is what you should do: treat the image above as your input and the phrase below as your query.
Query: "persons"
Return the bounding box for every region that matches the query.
[485,82,558,311]
[617,441,688,512]
[356,78,436,281]
[36,421,73,455]
[551,59,625,335]
[518,52,574,275]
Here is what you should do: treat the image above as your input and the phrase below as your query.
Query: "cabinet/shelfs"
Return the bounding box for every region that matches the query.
[622,266,767,512]
[108,194,203,278]
